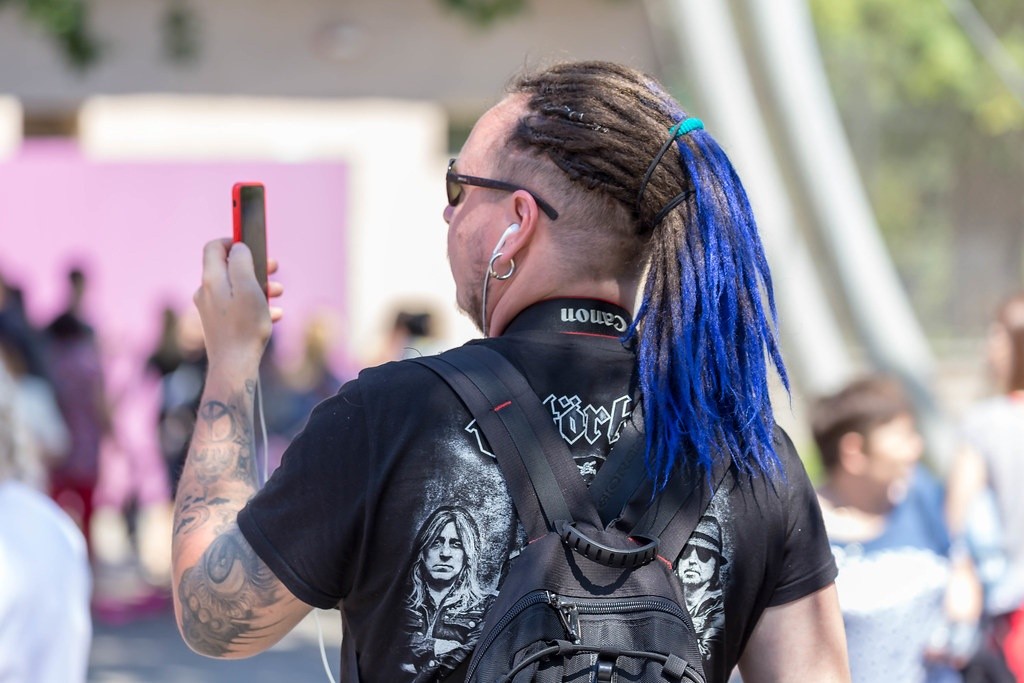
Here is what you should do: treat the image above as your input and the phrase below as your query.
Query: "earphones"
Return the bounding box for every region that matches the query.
[493,223,521,253]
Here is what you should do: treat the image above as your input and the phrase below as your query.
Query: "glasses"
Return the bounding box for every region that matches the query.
[445,158,559,222]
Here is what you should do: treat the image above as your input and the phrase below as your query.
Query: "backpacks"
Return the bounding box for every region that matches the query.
[410,343,732,683]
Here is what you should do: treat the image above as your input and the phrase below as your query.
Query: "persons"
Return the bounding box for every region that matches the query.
[252,300,442,497]
[807,286,1024,682]
[168,48,855,683]
[0,259,208,681]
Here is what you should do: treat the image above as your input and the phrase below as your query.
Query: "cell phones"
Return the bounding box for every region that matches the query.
[232,182,269,305]
